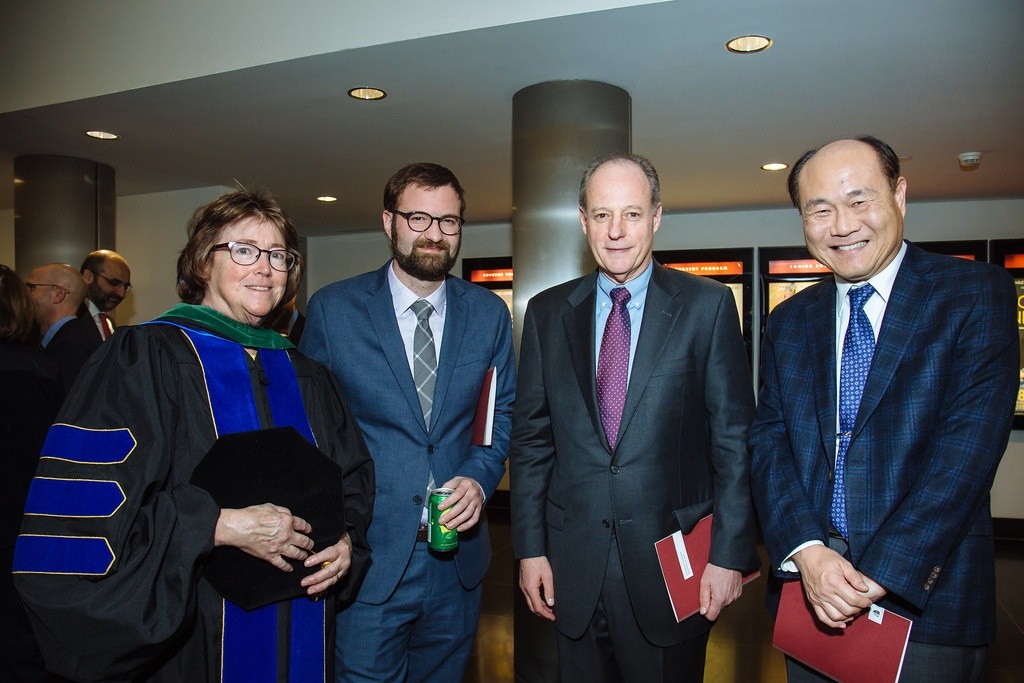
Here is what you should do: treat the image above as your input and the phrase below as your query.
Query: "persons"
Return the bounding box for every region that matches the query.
[20,261,99,421]
[73,249,132,372]
[510,154,765,681]
[748,136,1021,683]
[5,188,375,682]
[1,264,38,511]
[280,162,515,683]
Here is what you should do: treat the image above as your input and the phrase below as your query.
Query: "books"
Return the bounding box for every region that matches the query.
[655,514,762,624]
[475,366,498,449]
[772,577,914,682]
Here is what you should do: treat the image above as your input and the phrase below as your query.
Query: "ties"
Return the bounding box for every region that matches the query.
[411,300,439,432]
[597,287,632,455]
[827,284,877,544]
[98,313,112,341]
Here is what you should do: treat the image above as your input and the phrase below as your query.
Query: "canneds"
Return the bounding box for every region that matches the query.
[427,487,459,553]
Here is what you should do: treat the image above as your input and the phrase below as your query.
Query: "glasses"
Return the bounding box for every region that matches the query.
[388,208,466,236]
[90,269,131,290]
[24,282,70,295]
[210,240,299,273]
